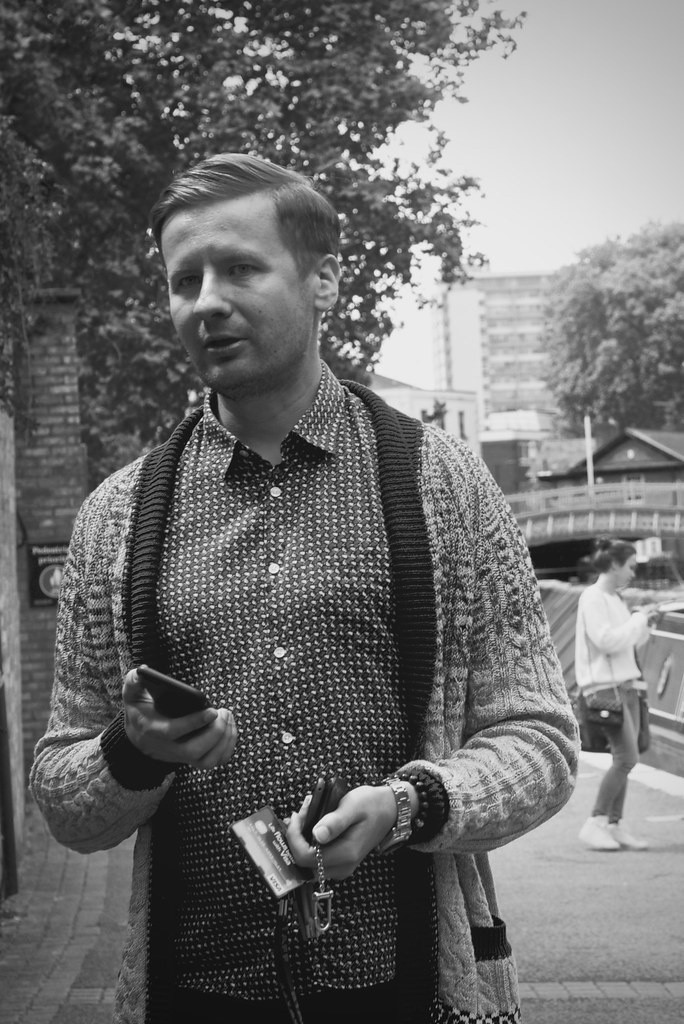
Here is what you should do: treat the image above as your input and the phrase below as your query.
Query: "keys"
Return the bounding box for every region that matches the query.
[303,774,347,846]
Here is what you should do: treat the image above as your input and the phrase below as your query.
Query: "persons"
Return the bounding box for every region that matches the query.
[29,154,580,1024]
[575,538,664,850]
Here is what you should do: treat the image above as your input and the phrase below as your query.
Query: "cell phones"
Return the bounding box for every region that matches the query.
[301,776,327,844]
[135,664,212,718]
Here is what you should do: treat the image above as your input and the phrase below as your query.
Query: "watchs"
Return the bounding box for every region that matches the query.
[375,776,411,855]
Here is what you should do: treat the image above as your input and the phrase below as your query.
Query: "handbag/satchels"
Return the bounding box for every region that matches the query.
[586,697,625,728]
[637,695,652,753]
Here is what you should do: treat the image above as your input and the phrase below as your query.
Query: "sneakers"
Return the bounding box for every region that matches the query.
[578,818,620,848]
[602,820,650,851]
